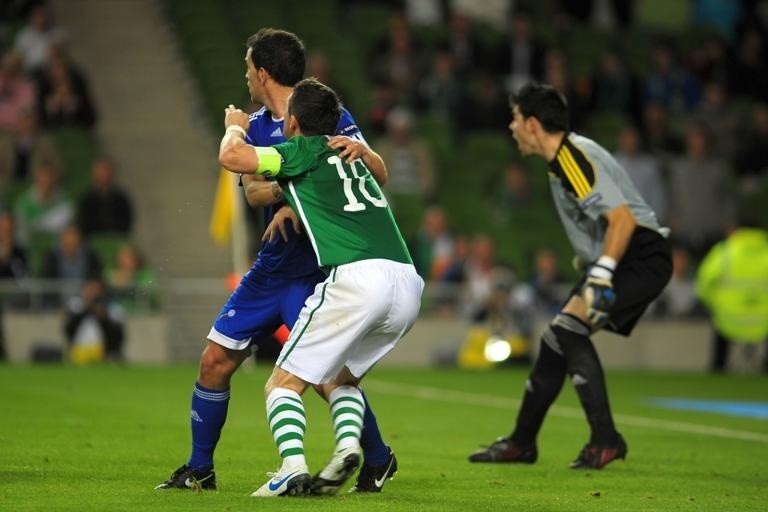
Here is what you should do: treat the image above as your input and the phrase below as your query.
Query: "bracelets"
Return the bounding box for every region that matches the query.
[224,125,247,138]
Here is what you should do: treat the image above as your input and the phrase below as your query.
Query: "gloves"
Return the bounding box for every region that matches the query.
[582,263,616,330]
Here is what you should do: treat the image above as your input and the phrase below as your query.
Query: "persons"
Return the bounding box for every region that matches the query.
[216,77,425,498]
[154,26,398,494]
[307,1,768,379]
[1,3,162,363]
[465,81,674,467]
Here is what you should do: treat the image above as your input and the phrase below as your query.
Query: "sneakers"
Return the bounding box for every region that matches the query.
[569,434,627,470]
[153,464,216,495]
[310,445,360,496]
[468,437,537,463]
[347,445,398,493]
[250,464,312,498]
